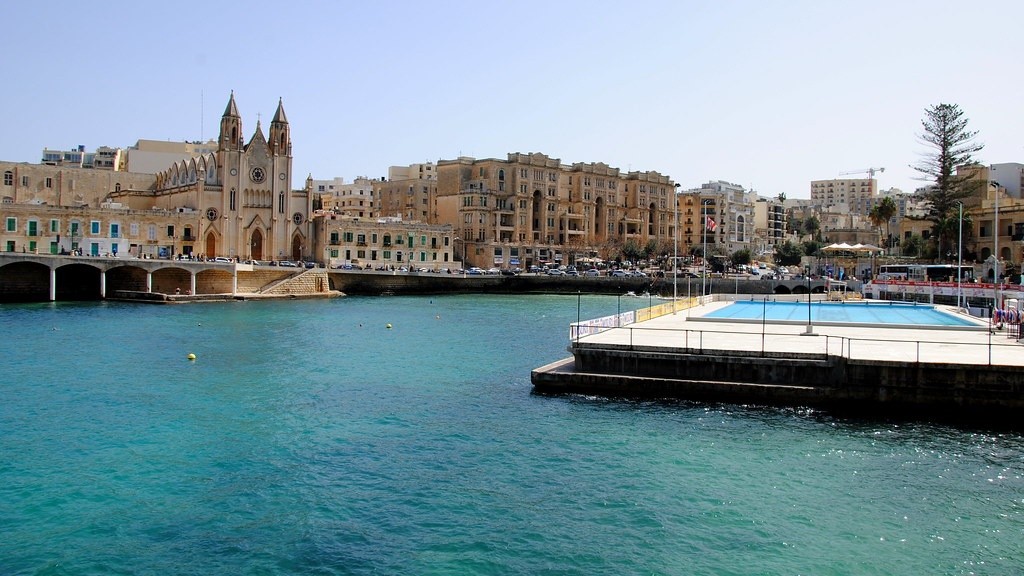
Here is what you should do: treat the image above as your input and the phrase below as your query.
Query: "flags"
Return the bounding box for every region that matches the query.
[706,216,718,231]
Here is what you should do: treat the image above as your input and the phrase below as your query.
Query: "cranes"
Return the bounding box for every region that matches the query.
[840,168,885,199]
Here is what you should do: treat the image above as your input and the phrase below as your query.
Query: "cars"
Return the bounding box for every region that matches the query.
[466,267,524,276]
[280,261,290,266]
[176,256,189,260]
[777,267,789,274]
[528,264,579,276]
[631,270,646,278]
[346,265,351,269]
[388,268,428,272]
[752,268,760,276]
[760,264,766,269]
[306,262,315,268]
[738,266,745,272]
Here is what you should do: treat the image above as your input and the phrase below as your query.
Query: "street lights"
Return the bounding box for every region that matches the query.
[454,237,466,275]
[989,179,1001,313]
[670,183,682,315]
[957,199,963,309]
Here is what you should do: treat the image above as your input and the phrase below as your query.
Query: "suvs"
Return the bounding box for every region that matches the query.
[612,270,632,277]
[582,269,600,277]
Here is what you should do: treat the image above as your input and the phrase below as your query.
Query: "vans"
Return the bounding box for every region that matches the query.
[215,257,230,262]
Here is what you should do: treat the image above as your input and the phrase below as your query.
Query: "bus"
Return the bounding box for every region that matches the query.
[878,264,975,287]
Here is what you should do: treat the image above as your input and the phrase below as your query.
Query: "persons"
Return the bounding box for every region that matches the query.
[61,245,207,262]
[23,244,26,253]
[235,255,452,274]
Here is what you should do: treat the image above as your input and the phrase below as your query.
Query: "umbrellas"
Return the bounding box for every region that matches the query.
[821,242,883,257]
